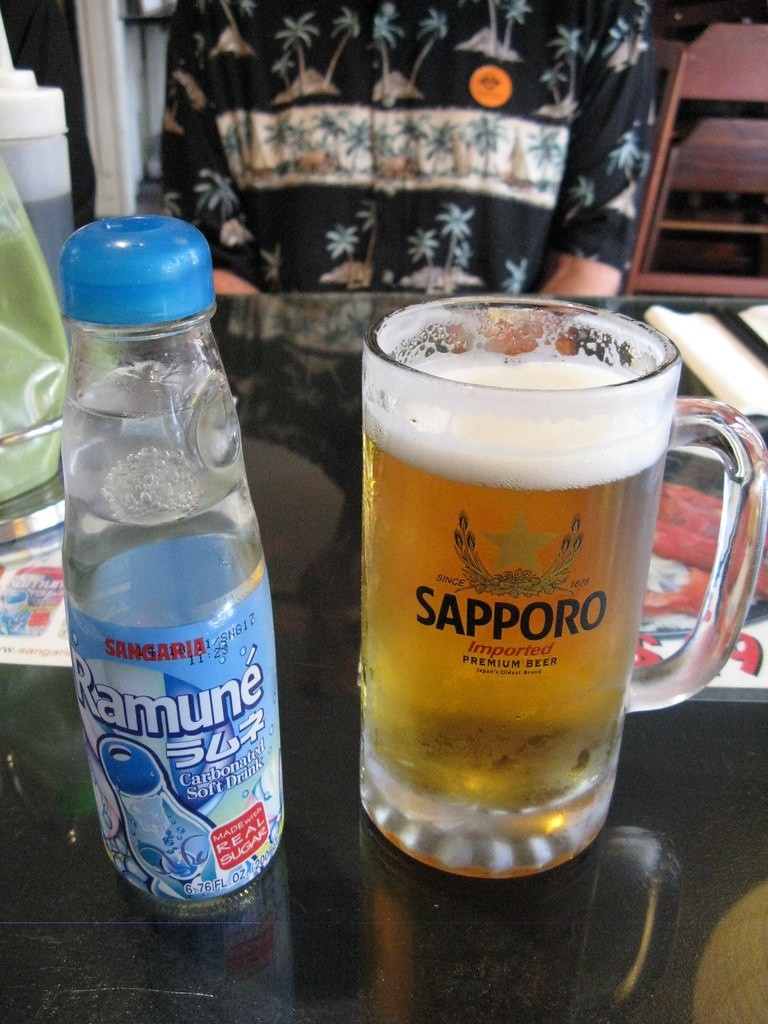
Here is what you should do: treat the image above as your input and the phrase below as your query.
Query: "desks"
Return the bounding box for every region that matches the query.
[0,294,768,1024]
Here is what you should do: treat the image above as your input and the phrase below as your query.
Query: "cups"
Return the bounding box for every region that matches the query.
[361,296,768,878]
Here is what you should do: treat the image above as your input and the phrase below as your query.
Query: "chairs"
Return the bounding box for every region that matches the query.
[623,26,767,294]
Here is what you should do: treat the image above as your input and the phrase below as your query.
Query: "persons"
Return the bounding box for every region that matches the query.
[157,0,659,298]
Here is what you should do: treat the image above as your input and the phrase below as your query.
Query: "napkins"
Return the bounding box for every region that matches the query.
[645,304,768,417]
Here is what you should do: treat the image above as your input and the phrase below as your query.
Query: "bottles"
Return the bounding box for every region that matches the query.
[61,214,283,918]
[0,70,73,305]
[0,155,72,516]
